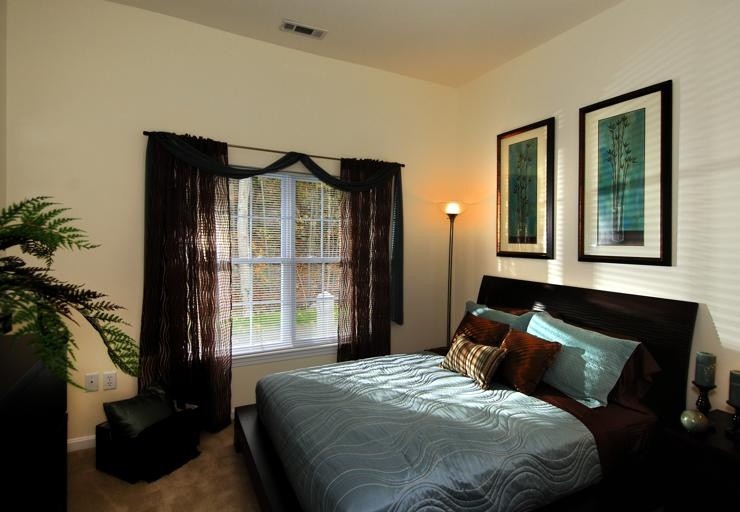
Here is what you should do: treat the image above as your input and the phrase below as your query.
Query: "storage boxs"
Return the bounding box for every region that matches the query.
[95,420,135,483]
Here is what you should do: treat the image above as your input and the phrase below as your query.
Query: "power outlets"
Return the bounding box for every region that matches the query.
[85,373,99,392]
[102,371,117,390]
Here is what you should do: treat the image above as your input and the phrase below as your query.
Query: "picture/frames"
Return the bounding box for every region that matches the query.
[495,117,557,259]
[577,77,674,268]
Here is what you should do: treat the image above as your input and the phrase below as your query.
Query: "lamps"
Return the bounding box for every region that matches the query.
[437,201,468,347]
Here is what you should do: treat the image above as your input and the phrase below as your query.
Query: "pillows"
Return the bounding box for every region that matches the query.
[497,327,562,396]
[439,333,508,390]
[507,308,642,408]
[450,311,508,347]
[465,300,517,326]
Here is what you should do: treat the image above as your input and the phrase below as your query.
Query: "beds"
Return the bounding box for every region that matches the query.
[254,275,699,512]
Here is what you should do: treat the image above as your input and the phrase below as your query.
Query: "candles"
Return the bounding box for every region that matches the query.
[694,350,716,386]
[729,369,740,405]
